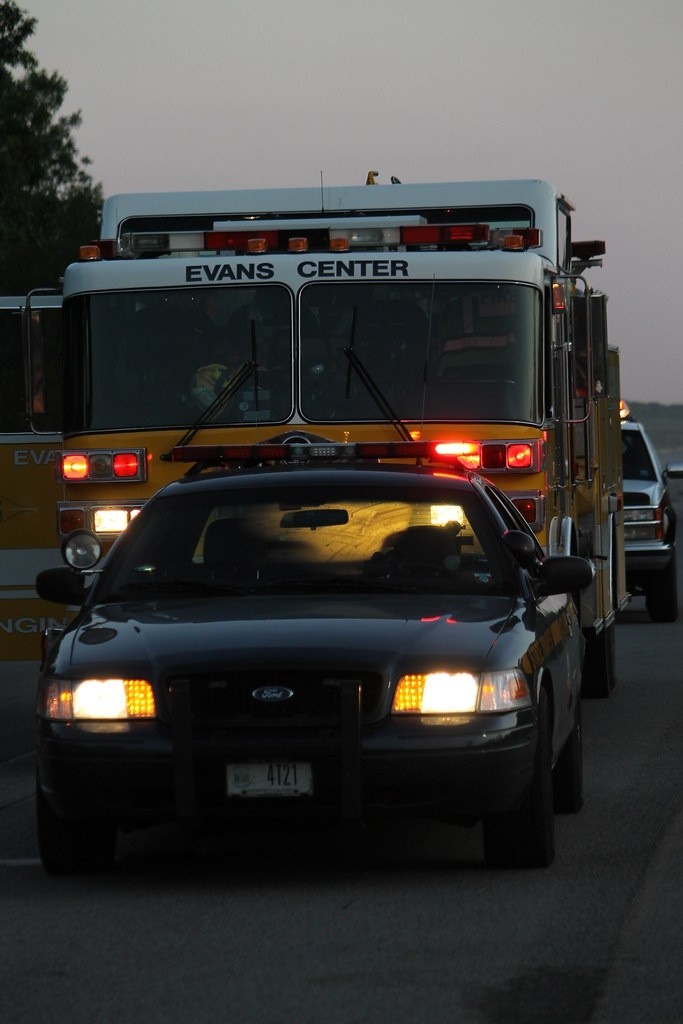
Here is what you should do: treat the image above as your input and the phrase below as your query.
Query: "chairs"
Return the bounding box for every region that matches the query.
[200,519,310,595]
[369,525,487,595]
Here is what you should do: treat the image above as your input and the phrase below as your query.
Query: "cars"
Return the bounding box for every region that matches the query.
[33,440,594,875]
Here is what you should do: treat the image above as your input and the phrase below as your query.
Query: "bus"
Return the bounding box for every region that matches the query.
[17,170,634,705]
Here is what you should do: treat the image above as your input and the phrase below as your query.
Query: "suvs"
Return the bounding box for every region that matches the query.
[616,397,679,625]
